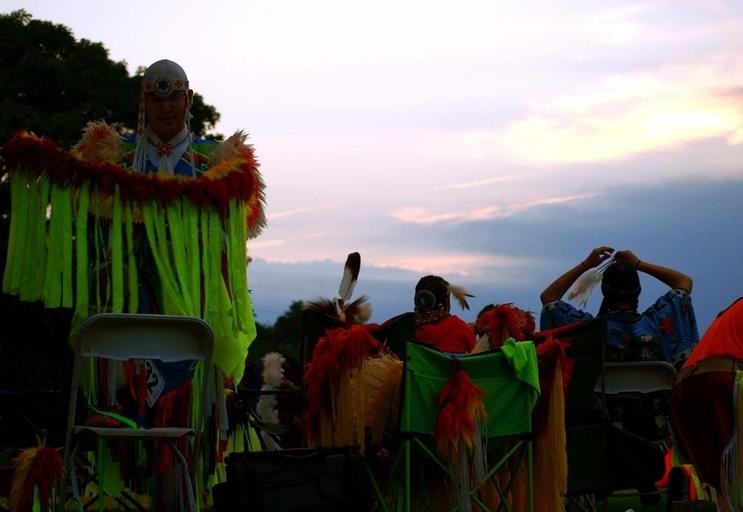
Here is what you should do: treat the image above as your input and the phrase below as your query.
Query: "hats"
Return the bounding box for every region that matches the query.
[140,59,190,97]
[413,276,472,325]
[601,257,642,296]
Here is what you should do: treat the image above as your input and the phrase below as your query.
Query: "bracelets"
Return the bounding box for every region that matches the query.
[634,259,641,268]
[582,262,589,270]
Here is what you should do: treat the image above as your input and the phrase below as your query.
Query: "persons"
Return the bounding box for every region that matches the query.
[0,59,267,508]
[375,274,477,361]
[538,245,701,483]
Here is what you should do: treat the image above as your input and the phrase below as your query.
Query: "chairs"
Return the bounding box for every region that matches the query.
[564,360,678,512]
[366,336,539,512]
[521,312,615,512]
[58,313,215,511]
